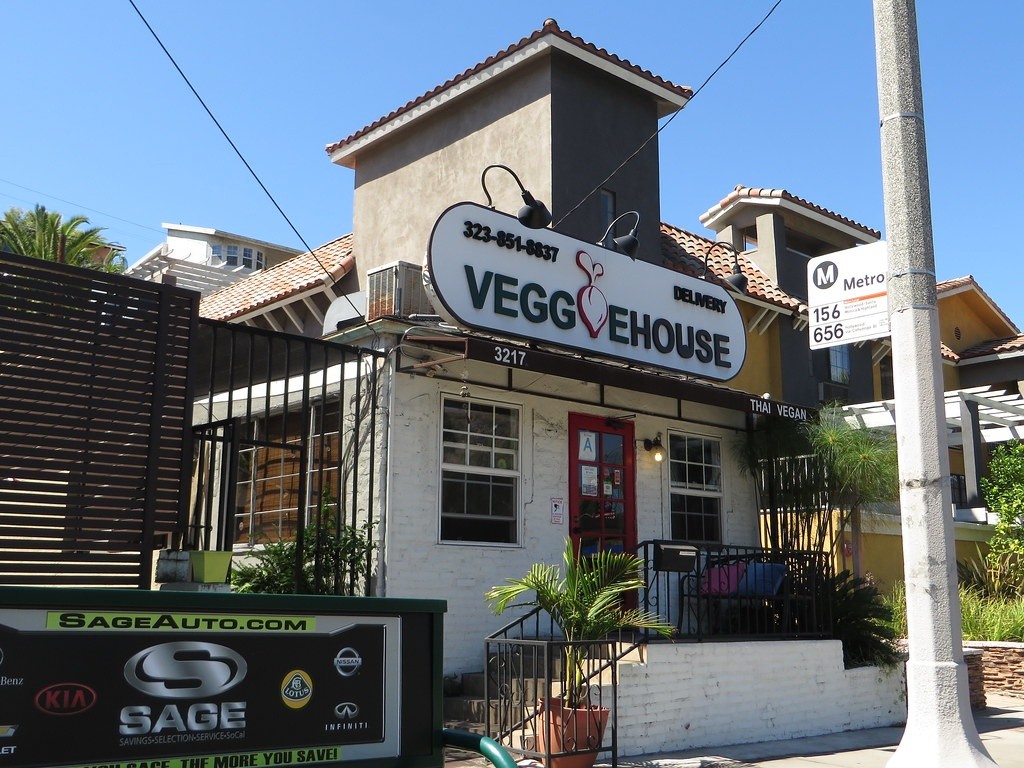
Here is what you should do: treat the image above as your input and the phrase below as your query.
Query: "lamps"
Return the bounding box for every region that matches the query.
[644,432,668,463]
[597,210,641,262]
[699,240,749,298]
[481,163,553,230]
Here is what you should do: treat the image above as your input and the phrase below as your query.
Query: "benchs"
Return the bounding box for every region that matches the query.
[676,552,817,631]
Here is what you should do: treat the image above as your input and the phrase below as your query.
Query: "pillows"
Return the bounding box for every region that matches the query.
[702,562,746,592]
[735,561,789,596]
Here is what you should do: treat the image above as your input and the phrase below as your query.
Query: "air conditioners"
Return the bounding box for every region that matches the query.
[818,381,850,406]
[365,259,436,319]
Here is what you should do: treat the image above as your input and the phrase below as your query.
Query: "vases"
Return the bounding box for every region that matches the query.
[190,549,234,584]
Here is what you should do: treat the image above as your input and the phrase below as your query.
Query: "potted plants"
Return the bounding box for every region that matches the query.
[486,534,680,768]
